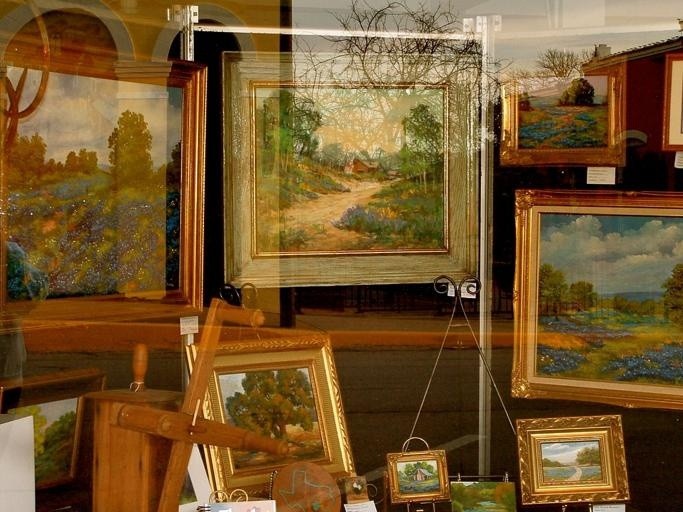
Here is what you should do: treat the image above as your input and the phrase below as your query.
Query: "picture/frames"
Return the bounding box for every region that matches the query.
[385,447,448,505]
[516,414,630,506]
[222,50,484,291]
[498,60,628,168]
[1,366,110,511]
[661,53,683,151]
[510,187,683,414]
[0,34,207,334]
[183,327,356,502]
